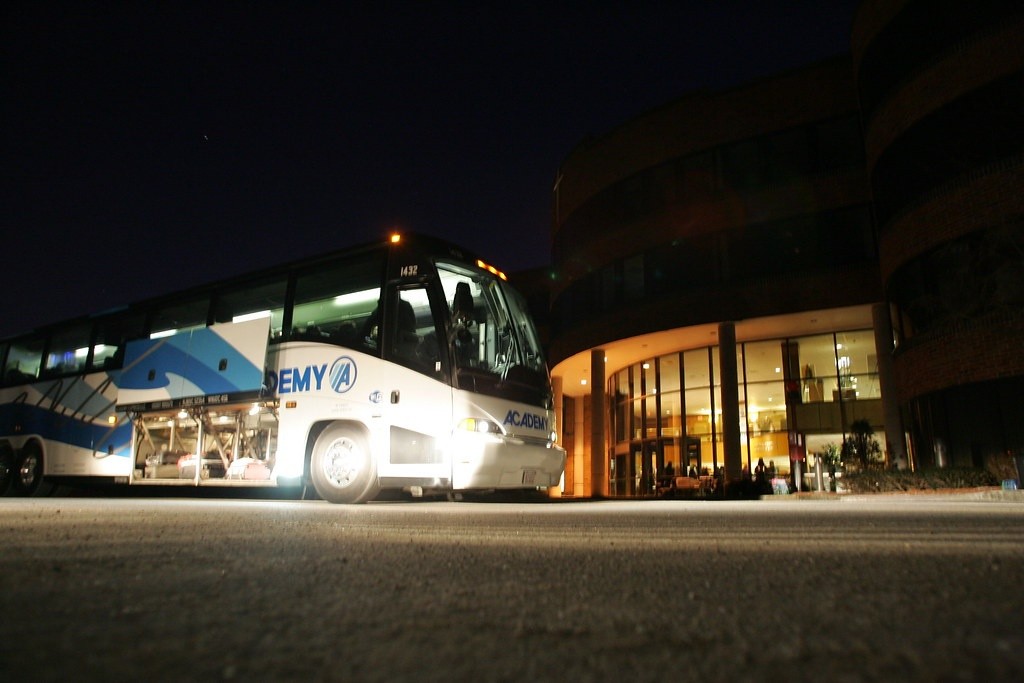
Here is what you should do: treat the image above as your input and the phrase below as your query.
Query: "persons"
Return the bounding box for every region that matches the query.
[358,283,476,372]
[638,456,776,498]
[893,454,908,474]
[269,322,358,348]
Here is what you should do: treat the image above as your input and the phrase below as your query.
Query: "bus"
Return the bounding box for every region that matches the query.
[0,236,567,506]
